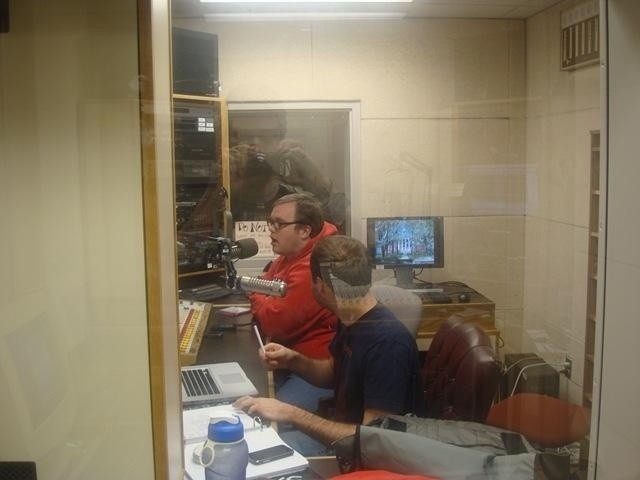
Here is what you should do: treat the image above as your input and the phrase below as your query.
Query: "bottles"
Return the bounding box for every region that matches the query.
[199,413,248,479]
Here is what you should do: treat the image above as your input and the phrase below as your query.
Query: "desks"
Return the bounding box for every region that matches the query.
[401,280,504,357]
[179,279,284,435]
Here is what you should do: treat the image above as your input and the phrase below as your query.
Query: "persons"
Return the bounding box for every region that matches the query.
[248,191,343,410]
[229,111,345,221]
[232,235,419,457]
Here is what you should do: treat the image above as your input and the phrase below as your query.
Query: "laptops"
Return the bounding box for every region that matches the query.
[180,355,260,406]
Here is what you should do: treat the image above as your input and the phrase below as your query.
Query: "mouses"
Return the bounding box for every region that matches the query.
[458,293,470,302]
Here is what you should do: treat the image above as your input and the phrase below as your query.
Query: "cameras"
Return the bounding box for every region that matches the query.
[246,151,282,176]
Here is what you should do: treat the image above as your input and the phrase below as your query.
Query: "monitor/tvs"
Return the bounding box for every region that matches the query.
[366,216,445,288]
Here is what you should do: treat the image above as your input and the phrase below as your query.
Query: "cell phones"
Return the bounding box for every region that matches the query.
[211,323,236,331]
[248,444,294,465]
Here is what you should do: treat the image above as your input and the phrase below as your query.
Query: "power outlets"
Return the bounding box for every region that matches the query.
[562,356,574,381]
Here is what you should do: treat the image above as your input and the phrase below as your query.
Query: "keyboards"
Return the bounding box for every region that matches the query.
[413,290,451,305]
[178,283,232,302]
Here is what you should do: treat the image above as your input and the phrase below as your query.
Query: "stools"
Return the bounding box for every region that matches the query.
[485,389,598,480]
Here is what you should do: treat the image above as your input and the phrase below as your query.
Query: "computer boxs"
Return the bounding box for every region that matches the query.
[505,353,560,399]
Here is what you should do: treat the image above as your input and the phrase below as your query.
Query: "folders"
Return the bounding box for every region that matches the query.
[181,404,309,480]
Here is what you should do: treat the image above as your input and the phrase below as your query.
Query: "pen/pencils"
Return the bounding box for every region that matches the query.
[254,324,265,353]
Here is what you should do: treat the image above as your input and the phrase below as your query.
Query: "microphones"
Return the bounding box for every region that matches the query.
[229,238,260,259]
[227,276,287,298]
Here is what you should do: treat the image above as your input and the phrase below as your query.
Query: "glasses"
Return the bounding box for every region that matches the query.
[265,216,301,232]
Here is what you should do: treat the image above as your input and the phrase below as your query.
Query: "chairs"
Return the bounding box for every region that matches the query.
[416,308,507,424]
[369,283,422,345]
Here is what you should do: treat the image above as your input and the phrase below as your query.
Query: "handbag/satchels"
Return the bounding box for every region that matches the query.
[362,413,539,462]
